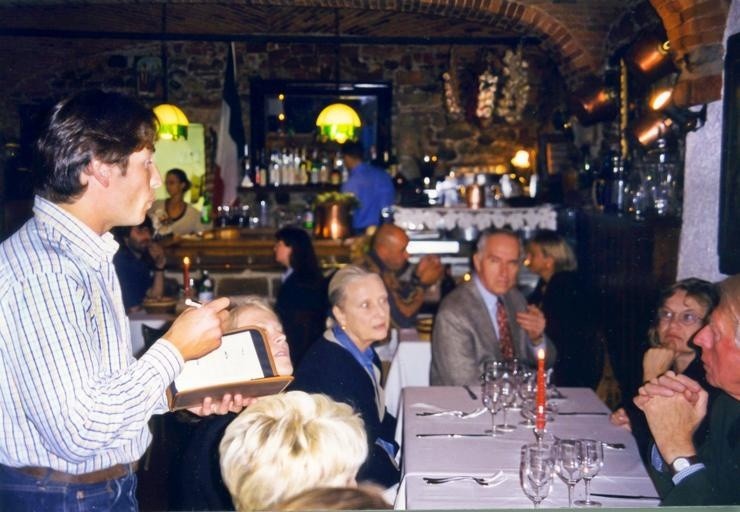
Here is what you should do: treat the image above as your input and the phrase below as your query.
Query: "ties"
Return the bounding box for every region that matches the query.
[495,299,516,371]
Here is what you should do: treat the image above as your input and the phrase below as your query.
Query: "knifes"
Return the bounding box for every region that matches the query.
[417,432,495,438]
[463,384,478,400]
[591,491,659,501]
[558,411,608,415]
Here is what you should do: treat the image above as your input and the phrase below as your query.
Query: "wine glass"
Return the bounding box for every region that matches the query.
[577,436,605,509]
[482,359,549,436]
[520,443,557,508]
[552,440,586,508]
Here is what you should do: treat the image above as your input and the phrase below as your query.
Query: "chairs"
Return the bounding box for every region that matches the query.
[126,260,286,356]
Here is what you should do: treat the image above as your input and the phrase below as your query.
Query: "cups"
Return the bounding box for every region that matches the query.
[599,148,681,218]
[416,313,434,341]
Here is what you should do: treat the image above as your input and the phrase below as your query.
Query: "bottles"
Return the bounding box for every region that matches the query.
[241,146,402,186]
[466,176,485,210]
[218,200,314,230]
[440,263,455,295]
[201,192,213,223]
[197,268,215,301]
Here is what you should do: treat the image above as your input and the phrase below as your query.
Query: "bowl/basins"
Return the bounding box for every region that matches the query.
[142,301,177,315]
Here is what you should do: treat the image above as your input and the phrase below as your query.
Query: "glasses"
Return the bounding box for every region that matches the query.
[657,306,708,326]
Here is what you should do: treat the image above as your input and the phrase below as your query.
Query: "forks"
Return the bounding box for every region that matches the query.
[550,384,568,399]
[423,468,508,485]
[416,408,486,418]
[550,434,626,450]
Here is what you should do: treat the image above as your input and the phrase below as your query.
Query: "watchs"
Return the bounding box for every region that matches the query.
[666,453,702,472]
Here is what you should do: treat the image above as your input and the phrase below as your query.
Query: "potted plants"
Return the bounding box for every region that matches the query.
[313,191,359,240]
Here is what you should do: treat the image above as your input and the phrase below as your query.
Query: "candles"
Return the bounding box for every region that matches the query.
[535,349,547,432]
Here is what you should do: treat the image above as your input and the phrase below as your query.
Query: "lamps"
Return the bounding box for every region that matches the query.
[148,2,189,142]
[312,7,364,146]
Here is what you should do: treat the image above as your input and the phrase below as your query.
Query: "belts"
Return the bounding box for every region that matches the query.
[15,460,140,487]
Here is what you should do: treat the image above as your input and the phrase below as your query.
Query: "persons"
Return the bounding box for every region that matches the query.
[148,168,202,241]
[340,140,396,234]
[164,280,294,512]
[611,280,724,429]
[114,215,168,317]
[220,394,371,509]
[267,487,394,512]
[273,227,328,340]
[633,274,739,508]
[429,231,559,392]
[328,224,444,340]
[524,238,592,374]
[299,265,405,484]
[0,99,255,512]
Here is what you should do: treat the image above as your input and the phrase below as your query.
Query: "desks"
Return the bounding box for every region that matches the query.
[387,202,560,235]
[387,260,540,389]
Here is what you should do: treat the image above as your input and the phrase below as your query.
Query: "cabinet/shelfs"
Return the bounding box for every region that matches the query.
[246,78,397,221]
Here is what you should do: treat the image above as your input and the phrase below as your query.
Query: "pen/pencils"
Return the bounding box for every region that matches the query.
[184,298,202,310]
